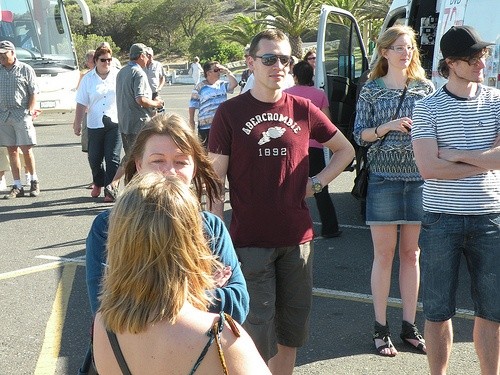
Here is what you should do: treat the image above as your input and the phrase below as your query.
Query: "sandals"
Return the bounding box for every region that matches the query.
[400,320,427,353]
[372,321,396,357]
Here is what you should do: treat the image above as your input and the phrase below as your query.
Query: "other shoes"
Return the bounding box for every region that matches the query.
[91,185,101,197]
[325,230,341,237]
[89,182,95,188]
[104,194,113,201]
[106,185,118,201]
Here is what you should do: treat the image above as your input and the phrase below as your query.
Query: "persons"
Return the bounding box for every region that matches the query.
[352,24,435,357]
[189,61,237,195]
[79,111,250,375]
[93,168,274,375]
[410,25,500,375]
[73,42,166,202]
[280,61,341,238]
[0,40,41,198]
[205,30,355,375]
[238,44,316,94]
[187,57,204,87]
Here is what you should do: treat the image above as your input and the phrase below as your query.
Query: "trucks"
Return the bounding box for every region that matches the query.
[314,0,500,176]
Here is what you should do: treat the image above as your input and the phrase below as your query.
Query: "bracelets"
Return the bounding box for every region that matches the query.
[225,71,232,76]
[374,123,384,140]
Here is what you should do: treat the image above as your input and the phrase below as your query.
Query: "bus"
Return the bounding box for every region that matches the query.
[0,0,91,112]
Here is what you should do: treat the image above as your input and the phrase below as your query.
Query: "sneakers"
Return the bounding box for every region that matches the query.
[3,185,24,198]
[30,180,40,196]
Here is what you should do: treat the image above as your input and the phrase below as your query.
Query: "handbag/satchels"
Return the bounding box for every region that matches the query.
[188,68,193,75]
[350,168,369,198]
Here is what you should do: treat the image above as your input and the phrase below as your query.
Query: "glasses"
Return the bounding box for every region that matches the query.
[209,68,220,72]
[454,50,485,65]
[251,54,291,67]
[307,57,317,60]
[386,45,415,52]
[97,58,112,63]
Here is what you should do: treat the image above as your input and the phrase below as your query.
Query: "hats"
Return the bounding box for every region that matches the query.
[130,43,149,54]
[0,41,14,53]
[440,26,495,58]
[145,47,154,56]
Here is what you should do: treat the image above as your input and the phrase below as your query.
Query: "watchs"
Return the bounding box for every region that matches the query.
[311,175,323,194]
